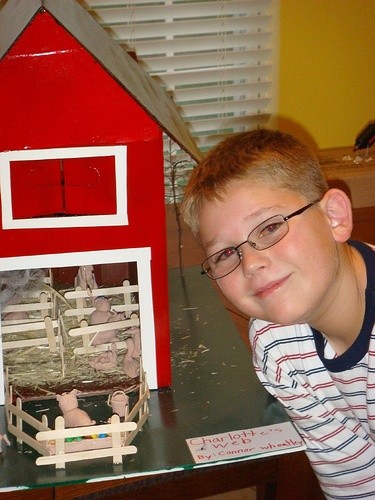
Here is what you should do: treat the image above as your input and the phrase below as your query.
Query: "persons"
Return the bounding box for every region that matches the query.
[176,128,375,500]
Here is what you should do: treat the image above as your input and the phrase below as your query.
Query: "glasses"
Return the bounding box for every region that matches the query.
[199,199,326,279]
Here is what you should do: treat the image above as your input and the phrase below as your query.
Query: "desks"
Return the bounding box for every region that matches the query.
[0,264,306,500]
